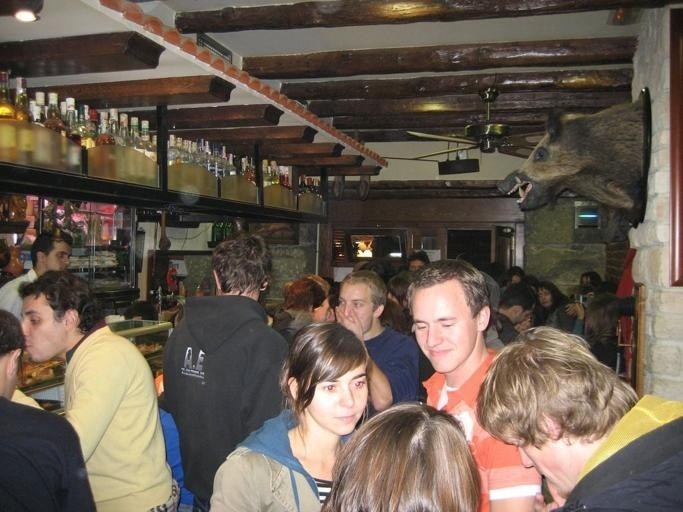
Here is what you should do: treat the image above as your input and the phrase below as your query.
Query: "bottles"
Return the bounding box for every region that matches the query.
[0,67,159,189]
[167,131,257,205]
[261,159,325,216]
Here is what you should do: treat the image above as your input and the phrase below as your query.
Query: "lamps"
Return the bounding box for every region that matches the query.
[0,0,43,24]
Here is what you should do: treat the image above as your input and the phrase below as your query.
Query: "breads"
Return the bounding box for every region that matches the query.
[18,356,62,387]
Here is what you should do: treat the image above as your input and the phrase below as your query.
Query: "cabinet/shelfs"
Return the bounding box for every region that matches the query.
[17,321,172,416]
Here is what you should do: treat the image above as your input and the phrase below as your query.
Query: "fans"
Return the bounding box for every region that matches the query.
[405,89,548,161]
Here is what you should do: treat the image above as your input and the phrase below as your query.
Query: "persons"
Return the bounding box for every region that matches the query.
[1,228,682,510]
